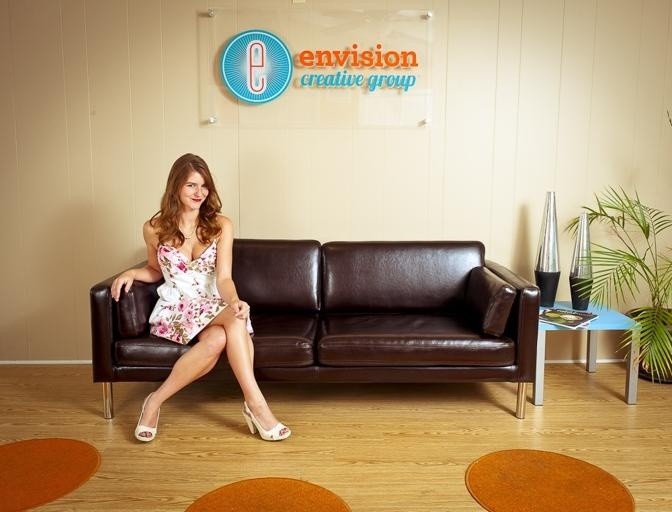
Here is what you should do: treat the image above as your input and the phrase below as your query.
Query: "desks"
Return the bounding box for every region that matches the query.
[534,301,642,406]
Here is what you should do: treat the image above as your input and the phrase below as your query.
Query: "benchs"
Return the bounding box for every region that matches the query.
[90,239,541,419]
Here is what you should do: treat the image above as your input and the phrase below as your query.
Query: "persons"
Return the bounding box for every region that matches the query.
[107,153,292,443]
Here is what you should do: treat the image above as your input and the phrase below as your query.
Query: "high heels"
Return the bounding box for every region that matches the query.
[242,399,292,442]
[132,391,161,445]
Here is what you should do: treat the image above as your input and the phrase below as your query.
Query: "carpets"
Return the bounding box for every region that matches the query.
[181,477,352,512]
[1,438,101,512]
[465,449,636,512]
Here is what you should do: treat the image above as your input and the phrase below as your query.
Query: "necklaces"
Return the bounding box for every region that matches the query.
[174,221,197,240]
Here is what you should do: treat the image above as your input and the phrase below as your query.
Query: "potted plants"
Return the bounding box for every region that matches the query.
[564,183,672,385]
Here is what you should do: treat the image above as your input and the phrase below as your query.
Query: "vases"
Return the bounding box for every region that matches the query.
[534,192,561,306]
[568,212,592,311]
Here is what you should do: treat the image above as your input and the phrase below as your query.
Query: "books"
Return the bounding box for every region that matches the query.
[539,306,599,331]
